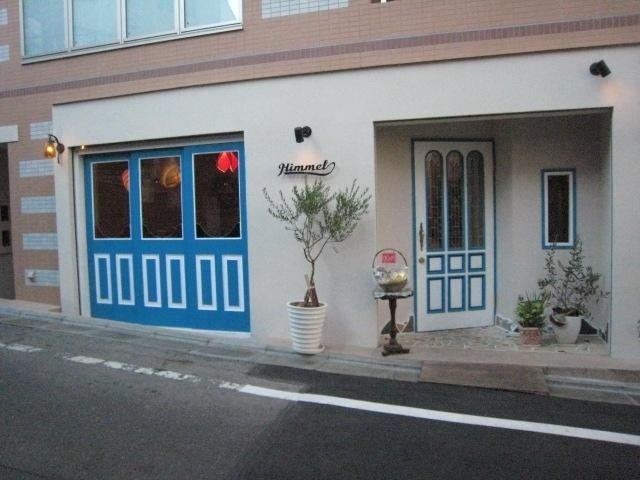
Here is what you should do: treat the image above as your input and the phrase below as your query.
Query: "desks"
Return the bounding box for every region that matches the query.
[373,288,415,359]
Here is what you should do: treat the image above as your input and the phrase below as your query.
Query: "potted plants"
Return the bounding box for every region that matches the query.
[263,179,374,356]
[514,235,602,345]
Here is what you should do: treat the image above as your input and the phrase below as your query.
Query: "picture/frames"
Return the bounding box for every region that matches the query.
[539,167,577,249]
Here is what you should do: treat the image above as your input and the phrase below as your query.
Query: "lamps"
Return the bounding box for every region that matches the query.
[293,124,313,143]
[44,133,65,165]
[591,59,611,79]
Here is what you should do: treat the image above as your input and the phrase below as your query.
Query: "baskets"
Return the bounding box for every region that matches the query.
[372,249,408,292]
